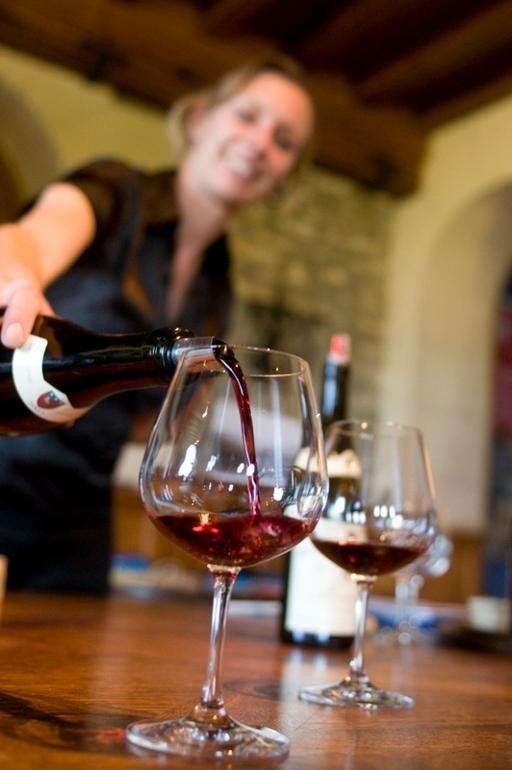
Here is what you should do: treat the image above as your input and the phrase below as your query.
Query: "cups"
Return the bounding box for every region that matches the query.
[126,342,332,763]
[296,419,443,711]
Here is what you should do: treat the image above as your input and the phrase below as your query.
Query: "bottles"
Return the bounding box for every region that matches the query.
[278,332,365,654]
[0,306,235,440]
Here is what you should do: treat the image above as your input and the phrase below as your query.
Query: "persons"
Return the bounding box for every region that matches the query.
[0,51,318,595]
